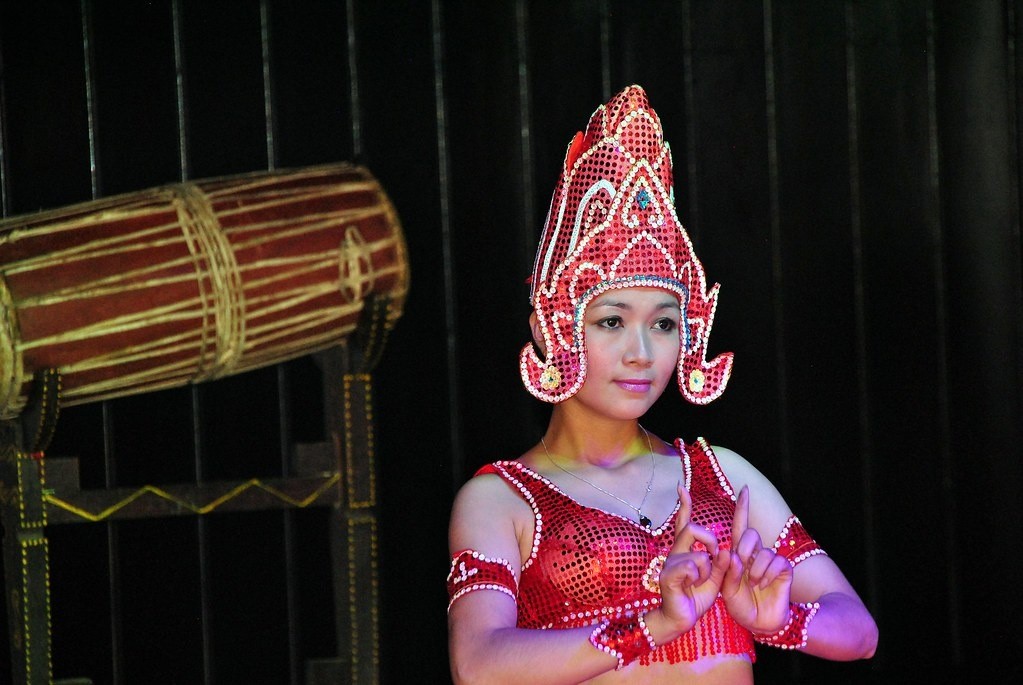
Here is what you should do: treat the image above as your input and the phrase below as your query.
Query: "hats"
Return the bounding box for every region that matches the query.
[520,84,735,405]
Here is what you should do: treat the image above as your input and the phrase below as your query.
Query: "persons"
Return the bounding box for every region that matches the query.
[446,83,880,685]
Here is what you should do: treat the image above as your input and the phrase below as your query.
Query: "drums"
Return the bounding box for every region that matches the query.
[0,159,413,421]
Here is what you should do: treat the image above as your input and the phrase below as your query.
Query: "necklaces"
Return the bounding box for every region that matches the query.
[541,423,656,529]
